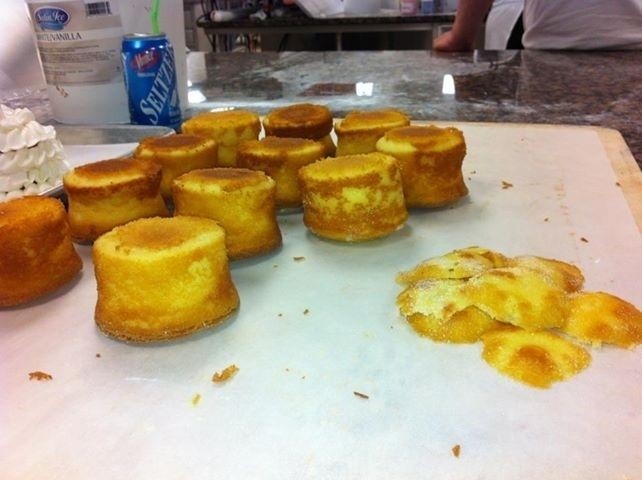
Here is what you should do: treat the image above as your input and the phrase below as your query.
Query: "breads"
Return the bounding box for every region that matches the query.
[179,110,261,166]
[296,152,408,243]
[236,137,324,203]
[334,110,410,157]
[0,196,82,308]
[375,125,469,210]
[263,104,336,158]
[63,157,170,246]
[136,134,218,199]
[92,216,240,342]
[397,242,640,389]
[171,166,283,258]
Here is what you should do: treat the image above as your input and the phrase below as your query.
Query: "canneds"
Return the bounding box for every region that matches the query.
[121,30,182,130]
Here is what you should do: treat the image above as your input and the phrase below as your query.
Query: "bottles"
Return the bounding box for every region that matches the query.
[421,0,433,16]
[398,1,416,17]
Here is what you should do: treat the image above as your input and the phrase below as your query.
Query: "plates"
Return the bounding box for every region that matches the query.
[38,141,141,199]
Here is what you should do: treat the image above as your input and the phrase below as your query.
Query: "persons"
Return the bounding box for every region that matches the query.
[432,0,642,54]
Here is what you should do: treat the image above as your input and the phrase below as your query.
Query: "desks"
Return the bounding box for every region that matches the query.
[197,12,471,53]
